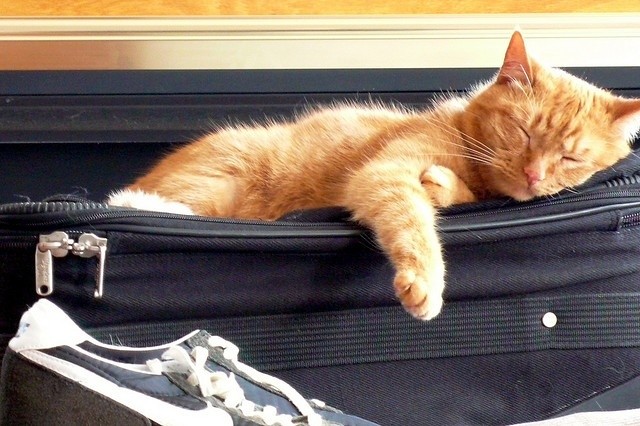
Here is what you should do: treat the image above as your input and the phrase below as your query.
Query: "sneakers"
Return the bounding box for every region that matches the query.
[2,296,374,424]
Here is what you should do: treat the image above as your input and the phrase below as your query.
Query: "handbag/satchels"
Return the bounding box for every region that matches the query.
[1,66,640,425]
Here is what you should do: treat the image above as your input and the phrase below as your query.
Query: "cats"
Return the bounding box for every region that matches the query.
[100,28,640,323]
